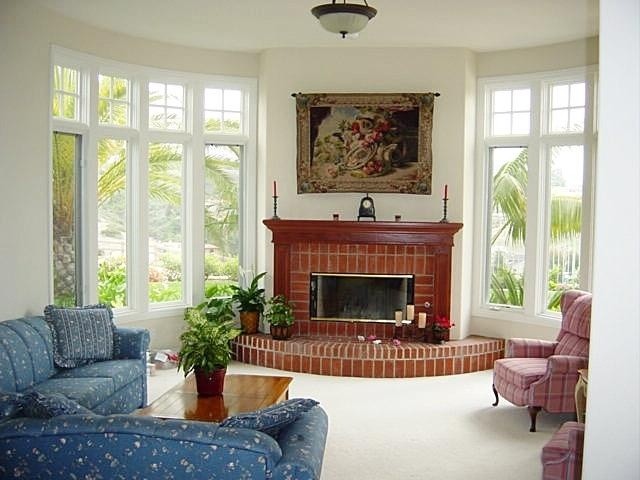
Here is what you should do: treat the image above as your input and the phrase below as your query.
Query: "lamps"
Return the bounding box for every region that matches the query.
[311,0,377,39]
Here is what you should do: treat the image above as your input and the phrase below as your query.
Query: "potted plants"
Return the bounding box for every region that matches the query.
[231,272,266,334]
[177,298,244,395]
[266,296,295,340]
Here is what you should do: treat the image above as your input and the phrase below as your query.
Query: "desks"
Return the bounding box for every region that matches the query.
[573,368,589,422]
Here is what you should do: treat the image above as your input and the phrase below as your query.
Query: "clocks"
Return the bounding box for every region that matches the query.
[357,193,376,221]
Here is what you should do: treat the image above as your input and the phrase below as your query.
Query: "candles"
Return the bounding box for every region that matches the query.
[274,180,277,196]
[407,305,415,321]
[418,312,426,328]
[395,311,404,327]
[444,185,448,198]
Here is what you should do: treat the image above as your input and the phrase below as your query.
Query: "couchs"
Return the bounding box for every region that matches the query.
[0,389,328,480]
[0,302,151,416]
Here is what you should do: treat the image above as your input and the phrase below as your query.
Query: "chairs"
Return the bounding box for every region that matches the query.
[492,289,592,432]
[541,421,584,479]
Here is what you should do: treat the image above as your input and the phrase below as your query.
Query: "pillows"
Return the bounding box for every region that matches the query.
[44,305,121,368]
[219,398,320,436]
[18,391,98,417]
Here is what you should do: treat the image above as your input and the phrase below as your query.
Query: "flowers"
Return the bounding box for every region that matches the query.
[432,316,455,332]
